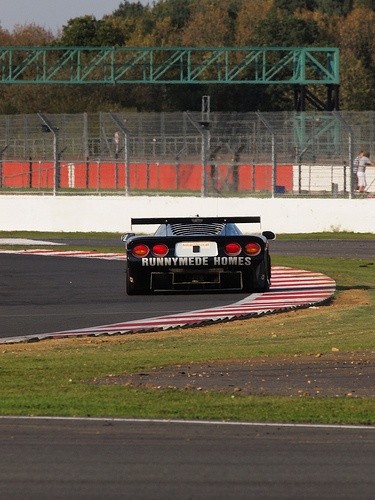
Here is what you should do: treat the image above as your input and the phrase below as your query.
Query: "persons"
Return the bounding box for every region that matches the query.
[353,150,374,194]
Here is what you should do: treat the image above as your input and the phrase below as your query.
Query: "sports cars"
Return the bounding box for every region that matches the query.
[121,214,276,292]
